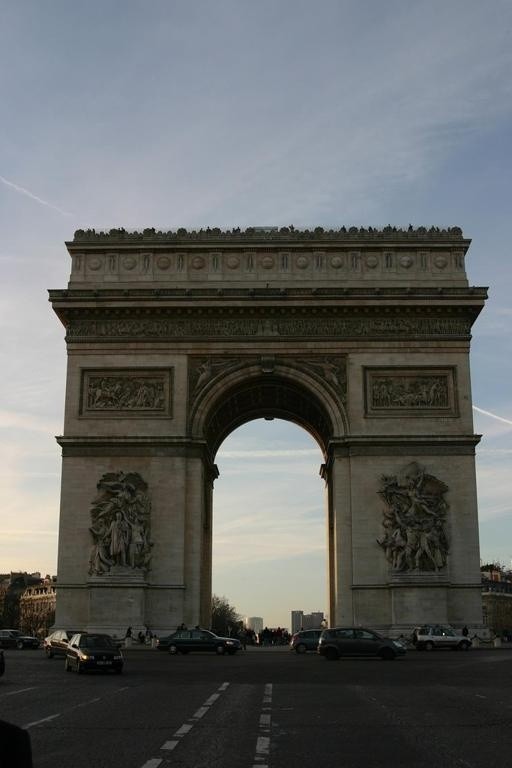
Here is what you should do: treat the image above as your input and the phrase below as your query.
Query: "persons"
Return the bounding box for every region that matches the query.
[89,377,156,407]
[194,358,227,393]
[90,482,162,574]
[238,626,248,650]
[124,623,188,649]
[374,375,447,407]
[305,358,349,396]
[462,624,469,637]
[373,483,450,574]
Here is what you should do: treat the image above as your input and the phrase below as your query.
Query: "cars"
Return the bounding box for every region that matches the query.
[318,626,408,660]
[64,633,124,673]
[44,629,88,658]
[0,629,39,649]
[155,628,240,655]
[289,629,324,653]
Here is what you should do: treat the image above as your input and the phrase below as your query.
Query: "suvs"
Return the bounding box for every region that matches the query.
[414,625,472,651]
